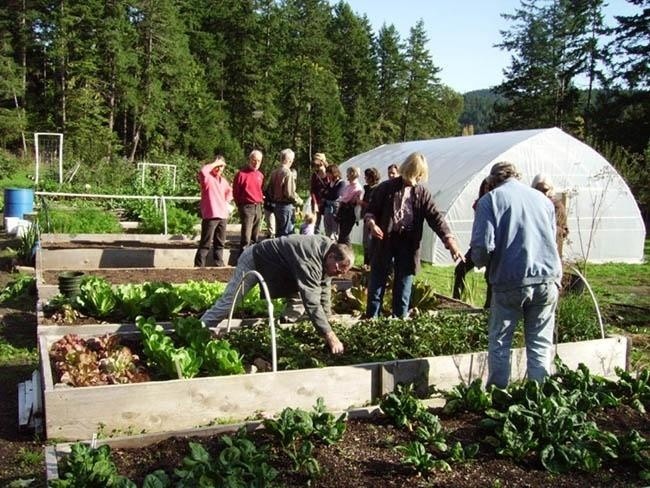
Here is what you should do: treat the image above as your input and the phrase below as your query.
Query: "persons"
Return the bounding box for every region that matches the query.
[452,179,494,309]
[470,160,564,391]
[531,172,570,259]
[300,150,400,245]
[200,234,354,354]
[232,150,265,257]
[194,156,235,267]
[266,149,303,238]
[365,152,466,315]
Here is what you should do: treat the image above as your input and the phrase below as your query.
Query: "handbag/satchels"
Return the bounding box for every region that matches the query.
[265,199,275,211]
[337,201,355,225]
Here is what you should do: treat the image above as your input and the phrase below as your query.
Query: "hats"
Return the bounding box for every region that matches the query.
[487,162,524,188]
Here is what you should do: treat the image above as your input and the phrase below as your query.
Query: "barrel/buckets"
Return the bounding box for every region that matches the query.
[3,186,34,218]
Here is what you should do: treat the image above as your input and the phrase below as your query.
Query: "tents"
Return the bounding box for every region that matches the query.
[304,127,646,267]
